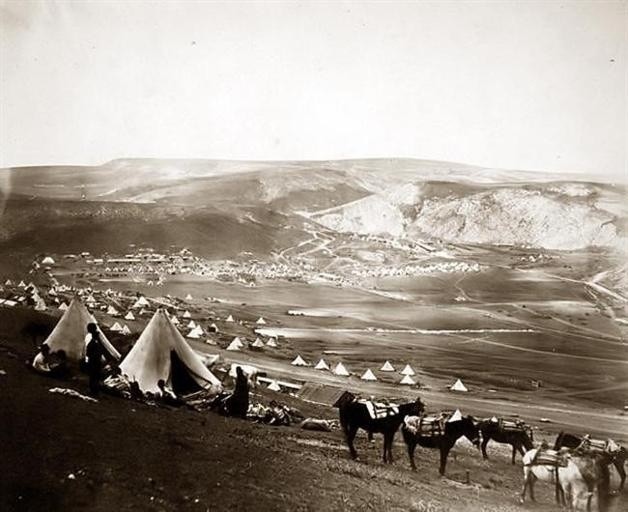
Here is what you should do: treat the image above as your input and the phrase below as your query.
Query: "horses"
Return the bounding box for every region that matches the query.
[400,414,479,474]
[518,431,628,512]
[342,395,428,463]
[474,416,533,465]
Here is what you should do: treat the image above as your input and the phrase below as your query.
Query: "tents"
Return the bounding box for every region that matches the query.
[183,309,277,350]
[33,296,122,378]
[449,379,467,391]
[293,356,349,376]
[362,360,417,386]
[6,280,180,325]
[103,307,222,402]
